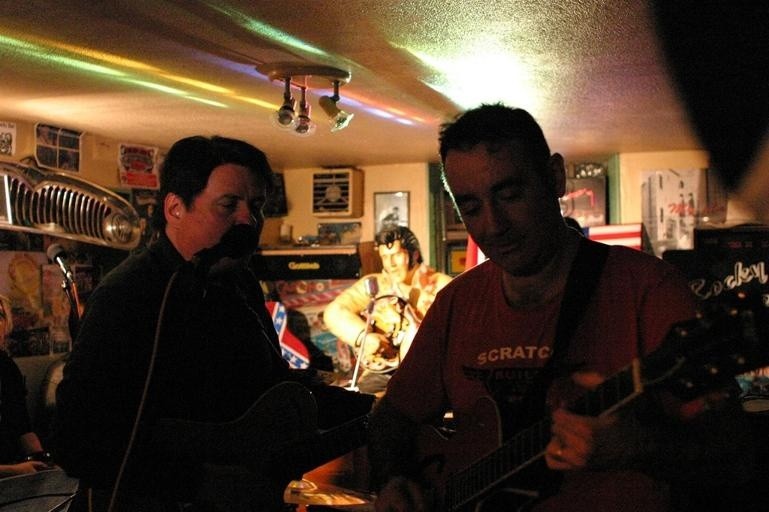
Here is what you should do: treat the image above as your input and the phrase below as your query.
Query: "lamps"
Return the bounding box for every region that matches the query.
[256,62,354,135]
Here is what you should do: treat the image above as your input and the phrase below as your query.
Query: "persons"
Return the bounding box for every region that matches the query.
[651,1,769,511]
[0,294,65,512]
[366,105,769,511]
[324,227,452,372]
[56,135,382,510]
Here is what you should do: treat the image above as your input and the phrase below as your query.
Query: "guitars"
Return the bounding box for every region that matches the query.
[370,293,762,512]
[352,296,423,373]
[95,378,374,512]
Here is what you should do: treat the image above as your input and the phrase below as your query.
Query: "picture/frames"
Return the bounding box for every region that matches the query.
[372,191,410,238]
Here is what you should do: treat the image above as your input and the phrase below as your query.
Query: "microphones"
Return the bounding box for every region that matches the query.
[47,241,76,284]
[188,223,261,273]
[367,275,382,302]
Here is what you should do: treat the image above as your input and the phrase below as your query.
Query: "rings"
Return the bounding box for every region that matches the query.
[554,443,570,459]
